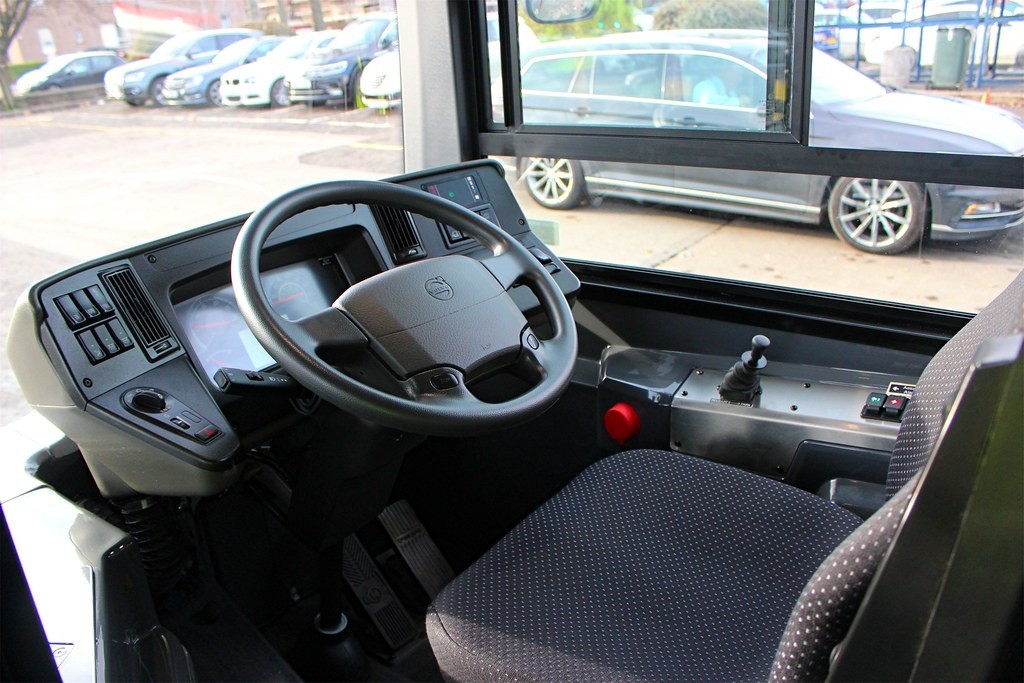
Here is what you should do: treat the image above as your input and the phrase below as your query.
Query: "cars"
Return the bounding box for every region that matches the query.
[864,4,1024,67]
[219,29,342,108]
[848,1,917,21]
[284,13,392,110]
[16,51,126,96]
[161,35,287,107]
[360,12,541,109]
[814,10,892,60]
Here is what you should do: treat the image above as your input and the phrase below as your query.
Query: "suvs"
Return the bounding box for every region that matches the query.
[517,29,1024,255]
[104,28,264,107]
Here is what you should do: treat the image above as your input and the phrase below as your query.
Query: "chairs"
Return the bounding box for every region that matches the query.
[425,267,1024,683]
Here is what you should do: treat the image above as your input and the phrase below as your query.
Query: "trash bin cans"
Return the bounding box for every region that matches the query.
[928,22,973,88]
